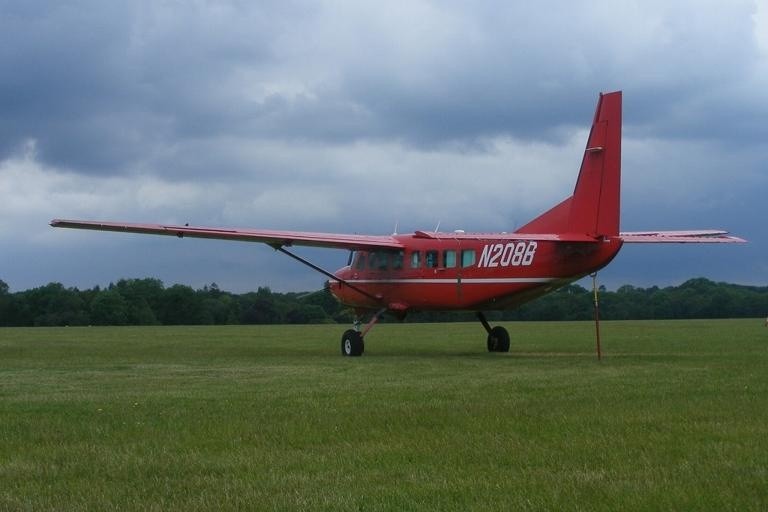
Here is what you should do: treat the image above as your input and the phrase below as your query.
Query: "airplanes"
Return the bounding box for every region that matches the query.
[47,88,744,360]
